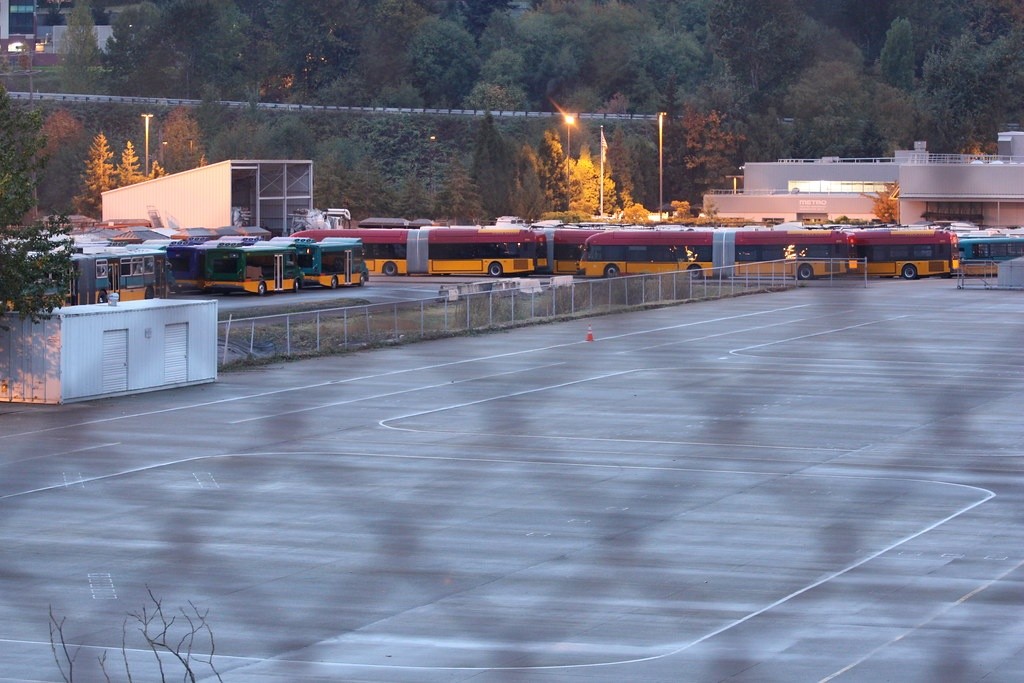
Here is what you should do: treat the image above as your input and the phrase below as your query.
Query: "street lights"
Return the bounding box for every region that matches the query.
[566,114,576,210]
[142,114,153,178]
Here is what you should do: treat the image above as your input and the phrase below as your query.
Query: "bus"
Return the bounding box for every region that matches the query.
[572,224,859,286]
[291,222,538,279]
[485,214,1024,282]
[198,243,303,298]
[0,208,267,313]
[253,237,370,288]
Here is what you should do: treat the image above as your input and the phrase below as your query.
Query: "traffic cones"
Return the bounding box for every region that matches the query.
[584,324,594,342]
